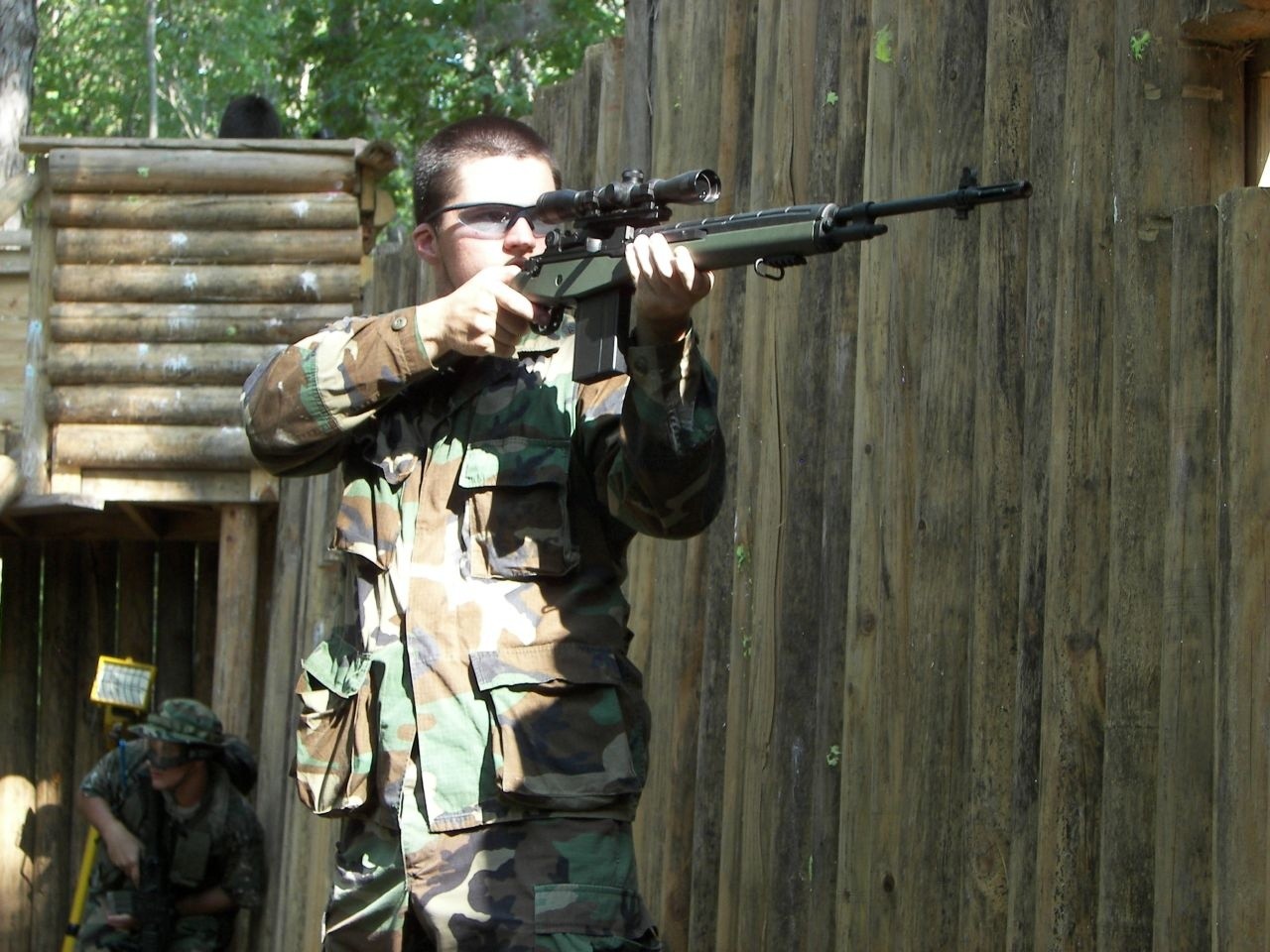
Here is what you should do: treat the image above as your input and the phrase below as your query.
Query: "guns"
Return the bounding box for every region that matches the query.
[107,771,175,952]
[502,163,1036,386]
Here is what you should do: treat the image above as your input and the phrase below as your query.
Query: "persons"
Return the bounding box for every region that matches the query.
[72,698,267,952]
[239,112,728,952]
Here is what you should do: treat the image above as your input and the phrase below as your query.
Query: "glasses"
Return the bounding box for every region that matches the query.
[423,204,555,236]
[145,742,218,769]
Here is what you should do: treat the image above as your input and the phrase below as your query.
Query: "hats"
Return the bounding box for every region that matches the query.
[219,732,257,796]
[128,698,227,745]
